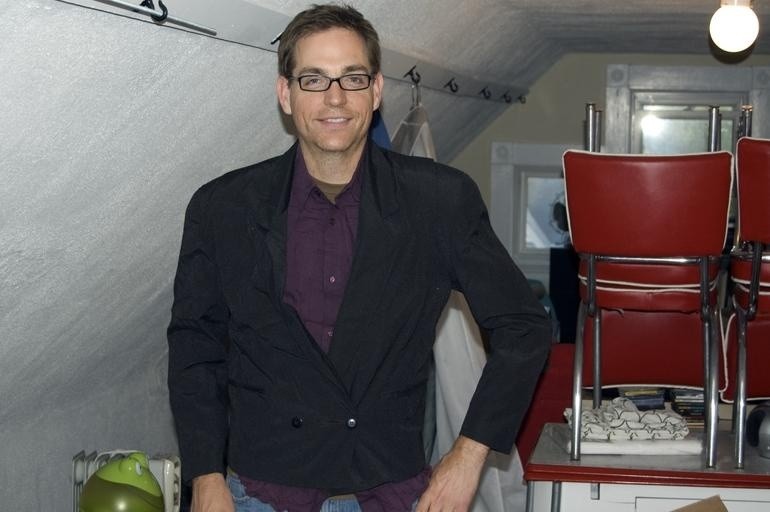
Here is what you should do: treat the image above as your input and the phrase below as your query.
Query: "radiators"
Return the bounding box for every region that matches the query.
[72,449,182,512]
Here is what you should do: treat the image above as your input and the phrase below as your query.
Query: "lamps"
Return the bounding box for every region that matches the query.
[709,0,760,64]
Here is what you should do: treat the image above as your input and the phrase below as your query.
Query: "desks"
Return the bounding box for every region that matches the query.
[526,421,770,512]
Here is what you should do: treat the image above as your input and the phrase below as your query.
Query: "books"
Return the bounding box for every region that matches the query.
[625,386,705,429]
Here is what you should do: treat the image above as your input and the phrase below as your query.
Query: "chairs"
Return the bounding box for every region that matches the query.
[562,148,733,468]
[720,135,770,468]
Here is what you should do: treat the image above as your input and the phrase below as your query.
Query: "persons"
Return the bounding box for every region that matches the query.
[165,5,552,510]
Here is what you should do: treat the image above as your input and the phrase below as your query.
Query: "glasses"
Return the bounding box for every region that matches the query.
[288,74,372,92]
